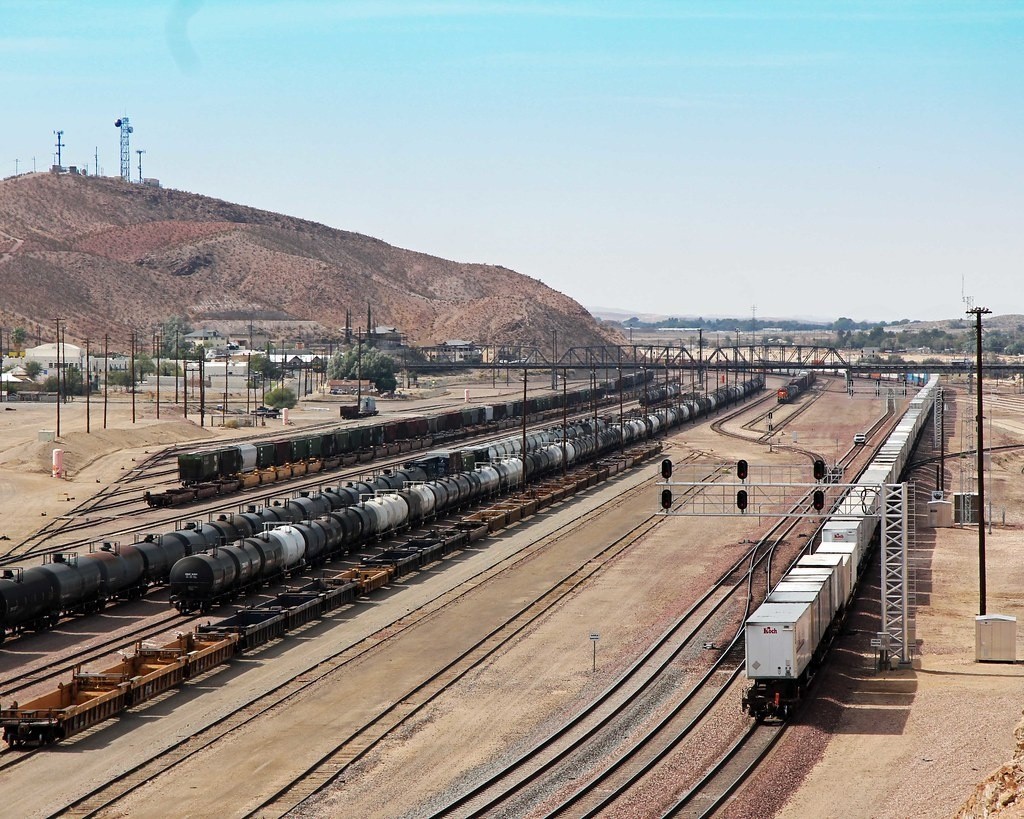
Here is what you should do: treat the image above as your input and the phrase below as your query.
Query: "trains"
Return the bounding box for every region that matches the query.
[742,373,941,723]
[1,369,765,634]
[142,371,661,507]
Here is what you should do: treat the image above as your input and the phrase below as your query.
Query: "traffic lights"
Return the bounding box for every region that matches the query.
[737,460,748,480]
[814,460,826,480]
[813,490,825,510]
[736,489,748,511]
[662,458,673,479]
[660,489,673,510]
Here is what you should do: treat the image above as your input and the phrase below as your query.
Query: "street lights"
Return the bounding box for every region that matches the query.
[966,305,997,620]
